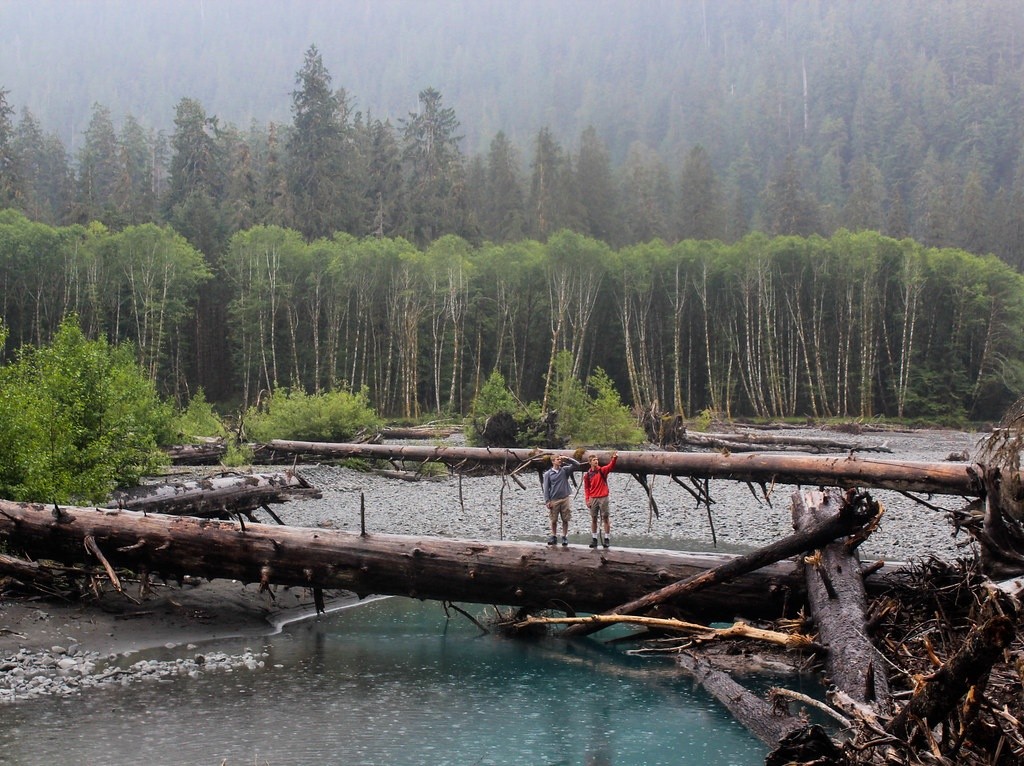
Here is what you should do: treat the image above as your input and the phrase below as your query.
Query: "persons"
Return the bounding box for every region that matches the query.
[583,451,618,548]
[543,453,580,546]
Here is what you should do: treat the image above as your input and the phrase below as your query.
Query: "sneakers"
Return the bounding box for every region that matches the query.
[603,538,610,547]
[548,536,557,545]
[589,538,597,548]
[562,536,568,545]
